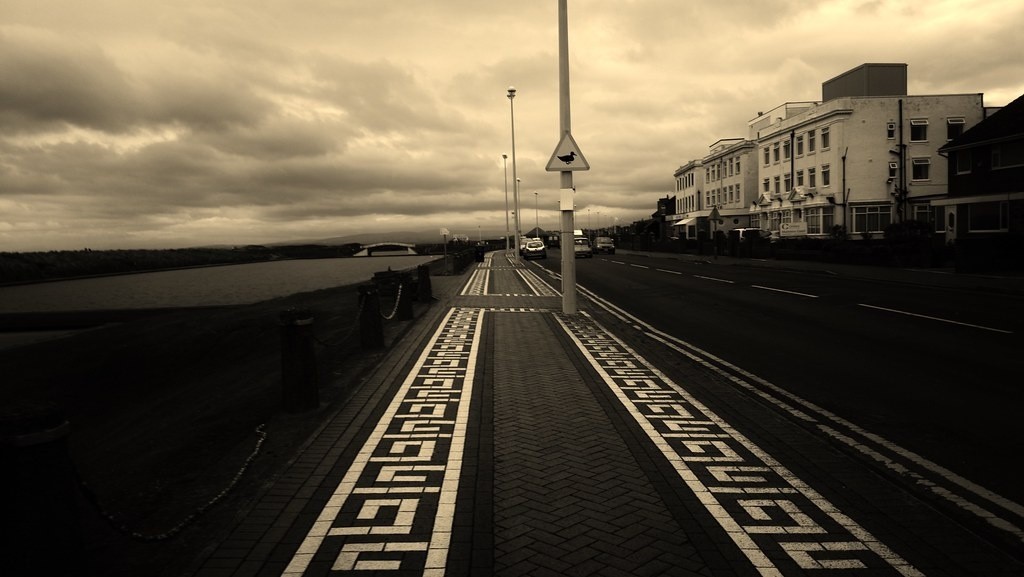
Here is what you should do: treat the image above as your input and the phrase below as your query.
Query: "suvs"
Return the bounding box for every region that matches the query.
[547,235,561,250]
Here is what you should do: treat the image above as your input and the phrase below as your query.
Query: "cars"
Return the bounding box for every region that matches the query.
[518,236,541,255]
[522,241,547,261]
[574,237,593,258]
[592,237,615,255]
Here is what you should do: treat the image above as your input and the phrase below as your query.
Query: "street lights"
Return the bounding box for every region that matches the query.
[558,200,561,234]
[506,87,521,265]
[573,204,577,230]
[503,152,511,252]
[534,192,538,239]
[516,177,521,232]
